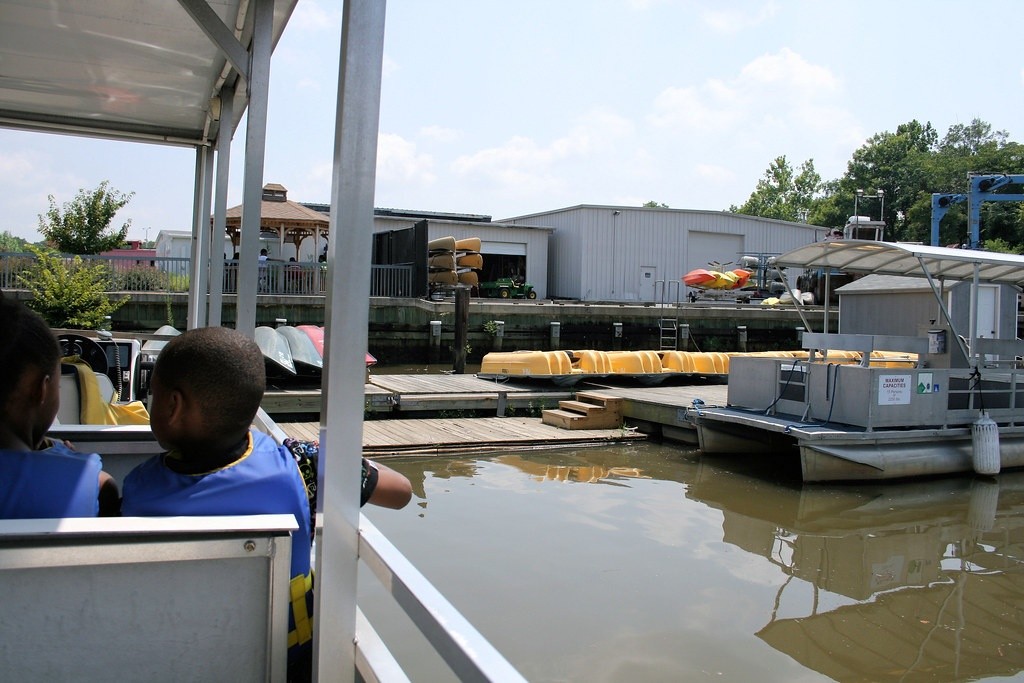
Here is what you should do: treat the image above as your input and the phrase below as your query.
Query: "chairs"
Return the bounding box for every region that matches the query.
[511,280,520,288]
[56,373,116,424]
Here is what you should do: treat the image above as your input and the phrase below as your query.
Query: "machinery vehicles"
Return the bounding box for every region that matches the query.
[795,215,886,305]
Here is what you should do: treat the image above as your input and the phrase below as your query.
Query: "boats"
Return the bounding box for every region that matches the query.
[428,236,484,287]
[253,323,377,376]
[680,238,1023,479]
[140,323,184,360]
[682,254,818,305]
[683,455,1024,683]
[475,342,919,386]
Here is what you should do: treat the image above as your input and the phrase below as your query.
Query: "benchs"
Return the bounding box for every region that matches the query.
[0,513,299,683]
[48,423,165,497]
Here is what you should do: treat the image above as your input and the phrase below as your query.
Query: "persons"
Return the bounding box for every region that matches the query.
[120,327,412,683]
[137,249,327,292]
[0,288,118,519]
[284,257,302,292]
